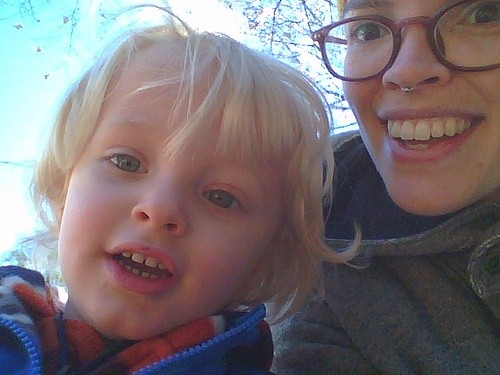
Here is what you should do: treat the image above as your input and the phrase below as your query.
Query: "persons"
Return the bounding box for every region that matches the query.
[265,1,500,375]
[0,0,362,375]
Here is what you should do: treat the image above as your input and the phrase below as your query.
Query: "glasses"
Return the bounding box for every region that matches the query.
[311,0,500,82]
